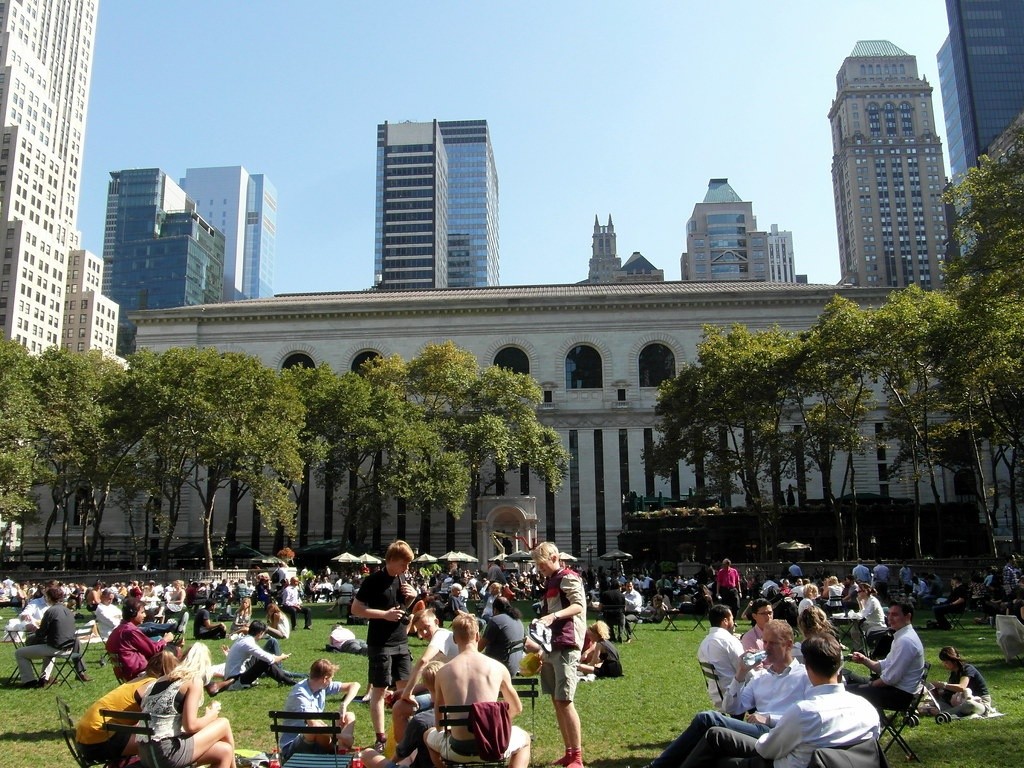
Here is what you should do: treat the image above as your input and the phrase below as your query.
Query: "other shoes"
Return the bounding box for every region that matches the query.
[75,673,92,682]
[626,638,631,642]
[40,677,60,685]
[374,740,386,754]
[178,612,190,634]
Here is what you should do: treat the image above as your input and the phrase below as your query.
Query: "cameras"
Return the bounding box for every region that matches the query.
[399,611,410,625]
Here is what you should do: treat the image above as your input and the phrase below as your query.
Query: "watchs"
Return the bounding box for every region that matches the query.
[554,611,561,620]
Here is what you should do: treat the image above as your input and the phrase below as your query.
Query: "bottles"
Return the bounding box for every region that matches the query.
[743,650,769,665]
[353,747,363,768]
[269,749,281,768]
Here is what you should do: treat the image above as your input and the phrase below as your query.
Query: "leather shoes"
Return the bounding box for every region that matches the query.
[206,679,235,697]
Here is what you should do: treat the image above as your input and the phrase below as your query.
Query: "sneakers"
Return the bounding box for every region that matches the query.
[526,618,553,653]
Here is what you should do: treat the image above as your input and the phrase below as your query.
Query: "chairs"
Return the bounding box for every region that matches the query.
[5,586,1024,768]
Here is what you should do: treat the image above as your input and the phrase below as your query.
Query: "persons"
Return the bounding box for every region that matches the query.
[0,558,1024,768]
[533,541,587,768]
[351,539,417,751]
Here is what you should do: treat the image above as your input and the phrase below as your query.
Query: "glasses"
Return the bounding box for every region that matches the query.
[757,611,772,616]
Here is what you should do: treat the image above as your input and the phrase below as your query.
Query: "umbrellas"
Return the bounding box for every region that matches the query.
[600,548,632,568]
[487,549,577,563]
[411,550,478,563]
[331,552,382,564]
